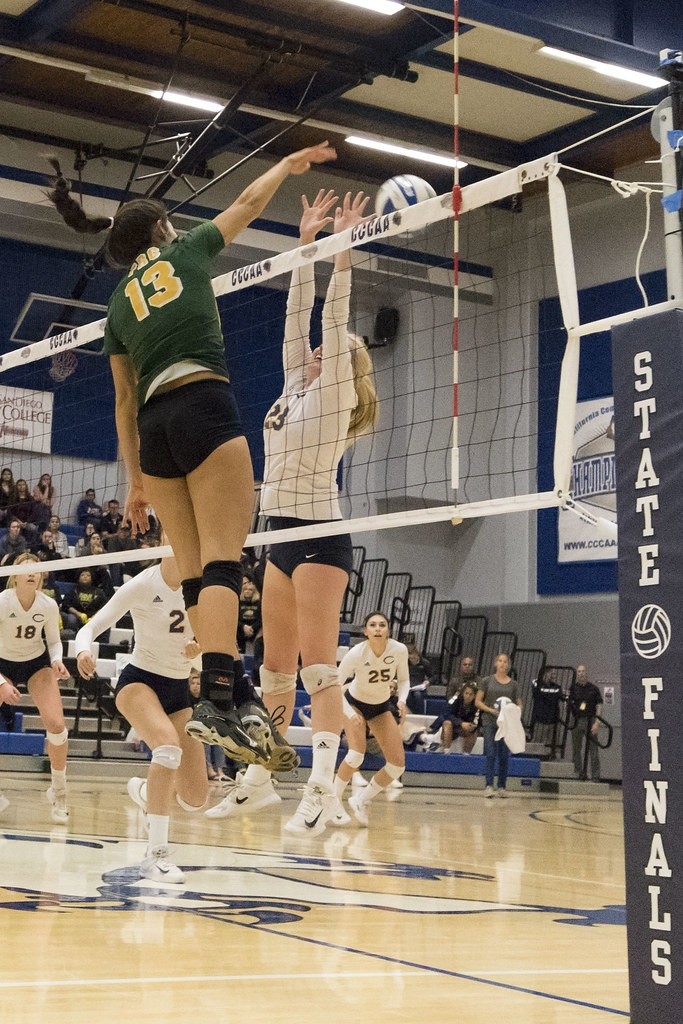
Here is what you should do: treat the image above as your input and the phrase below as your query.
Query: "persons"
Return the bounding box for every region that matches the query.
[40,138,340,773]
[474,653,524,798]
[426,657,481,734]
[205,187,382,836]
[0,552,71,826]
[529,665,563,744]
[72,530,207,887]
[0,467,432,786]
[569,663,604,783]
[443,683,483,756]
[330,611,410,827]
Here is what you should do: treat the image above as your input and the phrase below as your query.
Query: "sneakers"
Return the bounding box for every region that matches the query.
[348,794,372,828]
[47,787,68,825]
[139,844,185,884]
[184,700,270,765]
[127,777,150,834]
[284,778,340,838]
[352,772,367,787]
[389,779,403,787]
[331,800,351,825]
[0,796,10,812]
[204,772,281,821]
[236,702,297,772]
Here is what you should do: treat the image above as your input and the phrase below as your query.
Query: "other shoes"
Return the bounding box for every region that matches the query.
[498,790,507,798]
[485,786,495,798]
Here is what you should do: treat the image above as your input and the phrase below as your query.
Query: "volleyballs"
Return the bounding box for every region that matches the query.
[374,175,437,241]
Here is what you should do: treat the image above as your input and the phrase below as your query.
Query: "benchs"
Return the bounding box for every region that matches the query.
[0,522,611,797]
